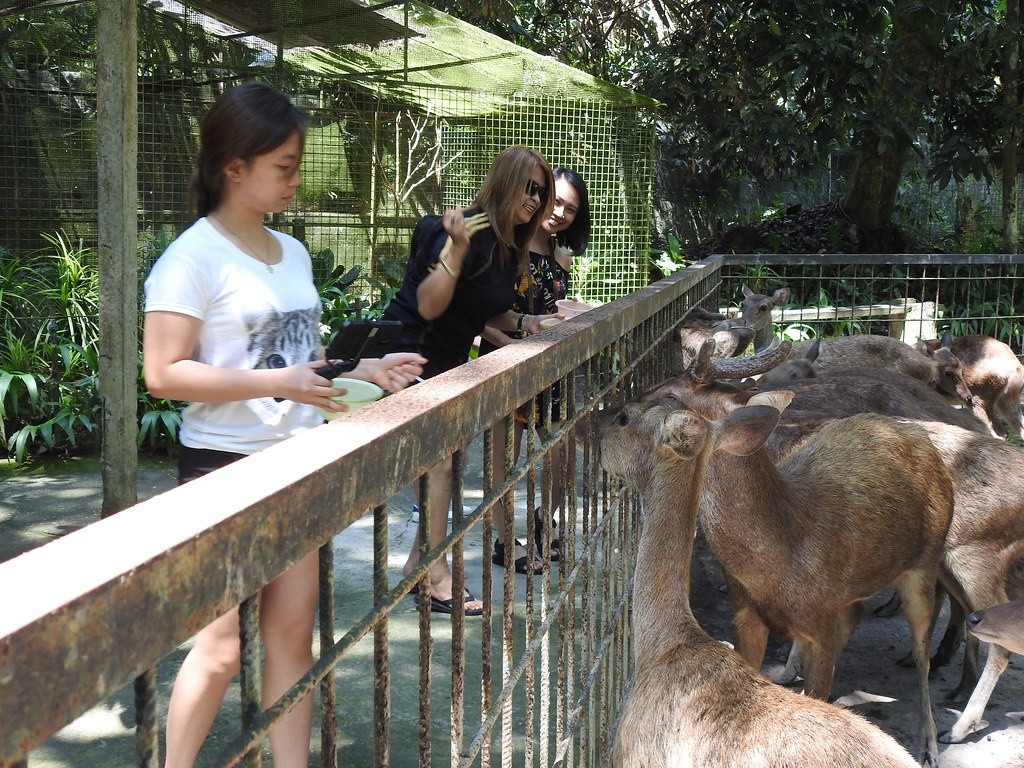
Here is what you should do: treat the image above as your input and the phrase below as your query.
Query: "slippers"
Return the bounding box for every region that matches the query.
[414,586,483,615]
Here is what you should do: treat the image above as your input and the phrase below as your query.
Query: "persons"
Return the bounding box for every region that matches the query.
[142,83,427,768]
[371,144,590,616]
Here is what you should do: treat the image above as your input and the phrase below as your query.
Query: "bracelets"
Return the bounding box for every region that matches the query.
[516,314,528,334]
[439,259,459,280]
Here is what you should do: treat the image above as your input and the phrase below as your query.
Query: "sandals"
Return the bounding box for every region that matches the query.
[535,507,561,561]
[492,538,543,574]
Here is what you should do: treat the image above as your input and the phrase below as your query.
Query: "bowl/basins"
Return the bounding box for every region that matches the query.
[320,377,383,421]
[540,316,572,331]
[555,300,592,317]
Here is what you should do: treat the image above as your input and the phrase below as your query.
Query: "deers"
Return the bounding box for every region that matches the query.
[561,287,1024,768]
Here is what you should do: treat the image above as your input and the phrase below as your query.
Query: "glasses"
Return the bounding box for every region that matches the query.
[525,179,546,202]
